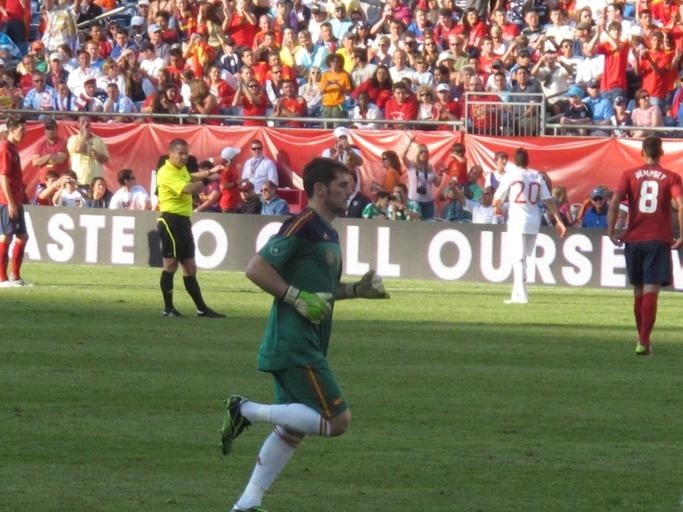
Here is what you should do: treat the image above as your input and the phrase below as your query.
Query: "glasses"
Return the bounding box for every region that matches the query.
[336,10,344,12]
[251,147,262,150]
[33,79,43,83]
[358,26,368,30]
[382,157,388,160]
[249,84,257,88]
[419,93,430,97]
[260,188,269,192]
[640,94,650,98]
[583,14,592,18]
[450,43,461,46]
[563,45,572,48]
[129,177,136,179]
[110,25,119,30]
[403,41,415,47]
[272,71,282,74]
[592,197,603,201]
[110,65,119,68]
[416,62,426,65]
[424,42,435,46]
[311,71,320,74]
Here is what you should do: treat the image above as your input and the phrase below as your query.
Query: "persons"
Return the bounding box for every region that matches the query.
[491,146,568,304]
[155,138,225,320]
[217,157,392,512]
[1,2,682,226]
[605,137,682,357]
[0,116,35,288]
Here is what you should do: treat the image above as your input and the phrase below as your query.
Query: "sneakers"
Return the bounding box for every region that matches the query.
[504,298,527,303]
[160,308,187,319]
[9,279,33,287]
[0,280,23,288]
[195,307,226,318]
[218,395,250,456]
[636,341,652,355]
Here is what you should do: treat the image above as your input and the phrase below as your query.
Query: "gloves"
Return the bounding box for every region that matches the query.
[282,284,333,325]
[345,268,391,298]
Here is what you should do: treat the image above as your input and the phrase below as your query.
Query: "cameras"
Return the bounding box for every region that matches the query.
[417,187,426,194]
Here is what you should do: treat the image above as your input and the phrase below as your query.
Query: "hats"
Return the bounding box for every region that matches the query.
[221,146,242,166]
[50,52,63,62]
[575,22,591,33]
[334,126,349,138]
[614,96,627,105]
[311,3,326,13]
[592,188,606,199]
[437,83,449,92]
[356,20,370,28]
[147,24,162,32]
[630,26,644,37]
[237,182,254,191]
[31,41,45,51]
[586,78,600,87]
[492,60,503,67]
[137,42,155,51]
[84,75,96,85]
[130,16,145,28]
[490,26,502,36]
[44,119,56,127]
[342,32,356,39]
[335,2,345,9]
[137,0,150,7]
[563,85,584,98]
[436,50,457,68]
[167,43,182,54]
